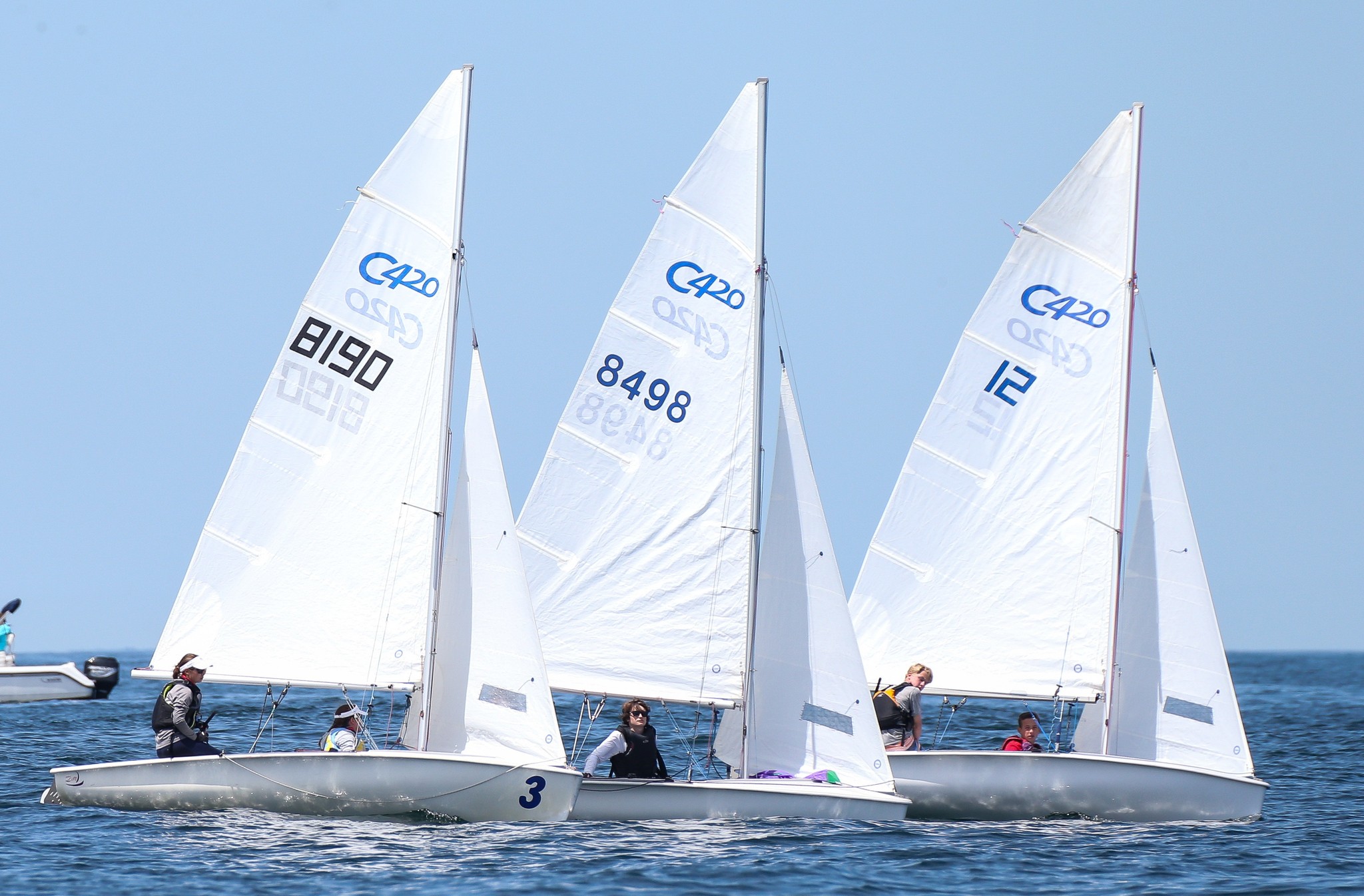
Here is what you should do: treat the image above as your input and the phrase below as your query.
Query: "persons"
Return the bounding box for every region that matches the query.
[873,664,932,751]
[1002,711,1044,751]
[318,704,366,752]
[152,654,225,758]
[0,613,11,651]
[581,697,671,779]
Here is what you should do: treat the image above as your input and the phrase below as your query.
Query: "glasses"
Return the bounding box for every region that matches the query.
[630,710,647,717]
[195,669,206,674]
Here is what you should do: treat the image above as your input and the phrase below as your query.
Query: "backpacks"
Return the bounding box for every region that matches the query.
[873,677,915,732]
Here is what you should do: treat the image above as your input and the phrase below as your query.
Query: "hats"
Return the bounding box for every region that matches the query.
[180,656,213,673]
[333,703,368,719]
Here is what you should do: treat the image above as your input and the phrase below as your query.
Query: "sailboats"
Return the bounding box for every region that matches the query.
[33,64,583,833]
[386,74,914,831]
[844,97,1277,828]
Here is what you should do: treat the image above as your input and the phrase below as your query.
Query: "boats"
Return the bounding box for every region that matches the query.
[0,647,121,703]
